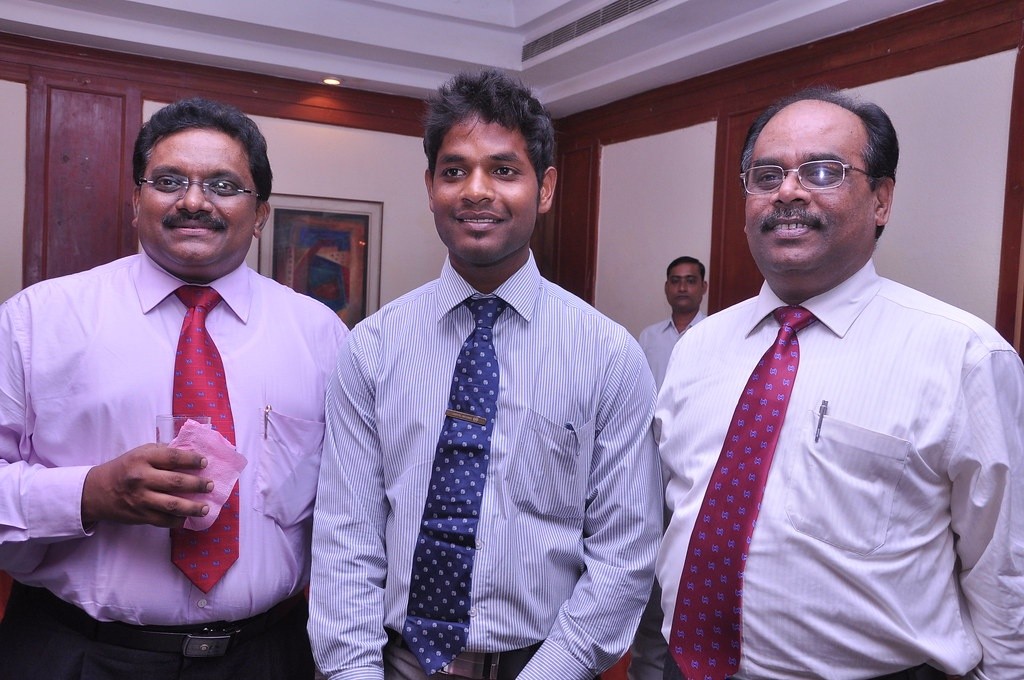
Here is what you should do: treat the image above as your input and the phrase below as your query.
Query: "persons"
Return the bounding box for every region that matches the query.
[0,101,350,680]
[306,72,664,680]
[650,97,1024,680]
[638,255,711,395]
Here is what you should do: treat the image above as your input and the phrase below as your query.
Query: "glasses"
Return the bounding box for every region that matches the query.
[139,172,262,199]
[740,160,878,195]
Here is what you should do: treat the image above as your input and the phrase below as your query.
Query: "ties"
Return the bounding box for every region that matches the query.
[403,297,509,675]
[170,285,239,594]
[667,306,816,680]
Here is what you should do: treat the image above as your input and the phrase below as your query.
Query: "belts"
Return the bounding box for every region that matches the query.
[32,587,304,658]
[384,628,545,680]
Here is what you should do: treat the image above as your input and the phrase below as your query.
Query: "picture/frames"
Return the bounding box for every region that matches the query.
[257,193,384,330]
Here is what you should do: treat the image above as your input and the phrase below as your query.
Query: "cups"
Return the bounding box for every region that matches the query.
[155,414,211,449]
